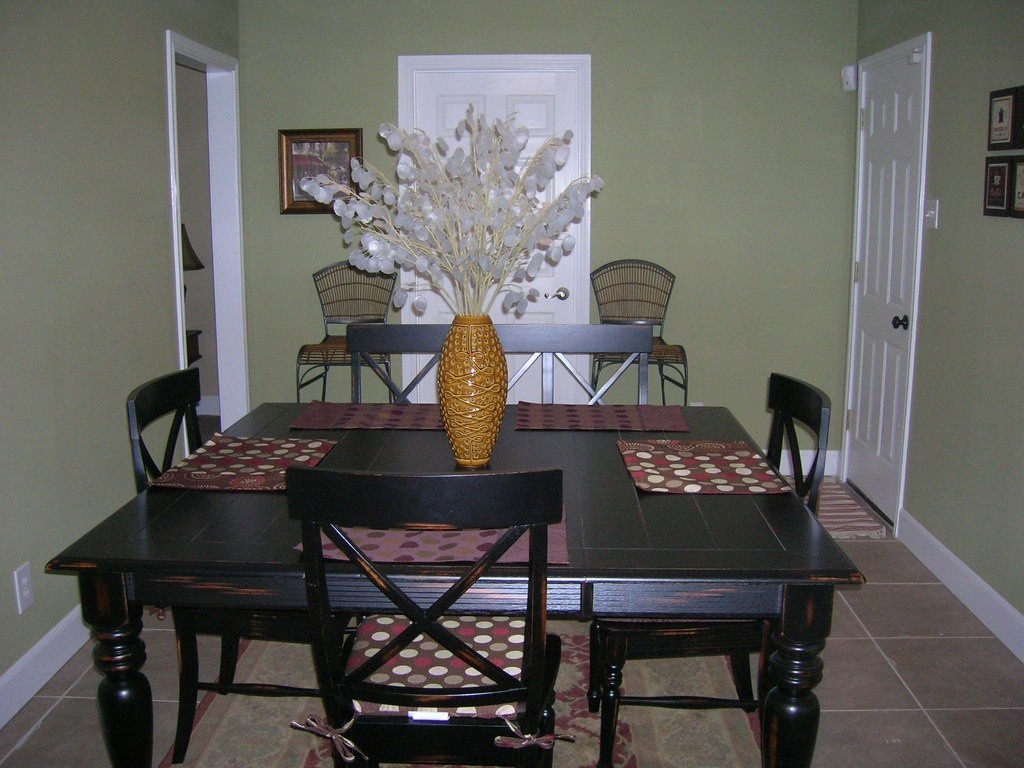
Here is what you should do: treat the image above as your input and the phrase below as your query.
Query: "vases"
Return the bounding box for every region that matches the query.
[437,314,508,468]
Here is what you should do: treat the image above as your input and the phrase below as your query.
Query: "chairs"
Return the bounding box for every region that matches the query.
[587,371,832,768]
[126,367,366,764]
[590,258,689,408]
[297,260,394,405]
[346,322,652,406]
[282,464,564,768]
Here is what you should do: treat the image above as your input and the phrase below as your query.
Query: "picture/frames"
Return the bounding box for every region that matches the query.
[277,128,363,214]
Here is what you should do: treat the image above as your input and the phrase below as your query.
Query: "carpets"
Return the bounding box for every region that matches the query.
[156,623,764,768]
[783,475,886,540]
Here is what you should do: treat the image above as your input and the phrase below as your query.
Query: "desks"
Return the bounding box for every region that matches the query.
[44,402,867,768]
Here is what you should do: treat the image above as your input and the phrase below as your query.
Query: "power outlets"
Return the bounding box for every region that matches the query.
[13,562,33,615]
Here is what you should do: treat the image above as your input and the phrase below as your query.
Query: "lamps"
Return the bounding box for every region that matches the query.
[182,224,205,300]
